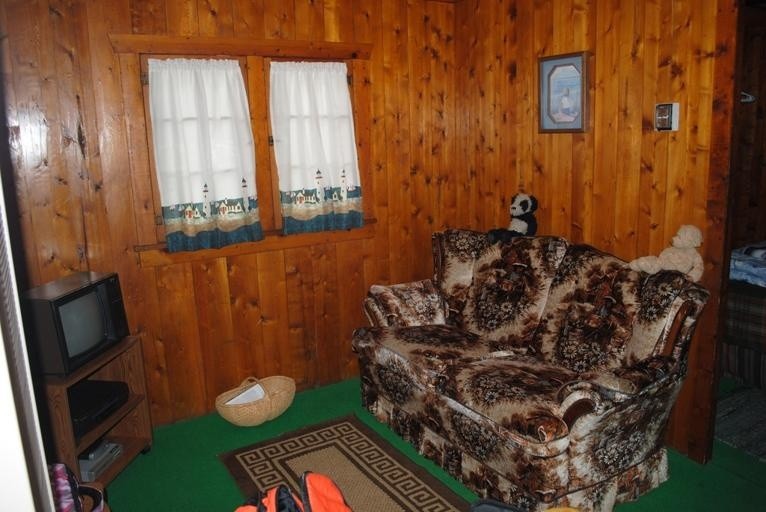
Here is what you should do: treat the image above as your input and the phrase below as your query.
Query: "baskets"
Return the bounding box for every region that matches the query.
[215,375,296,427]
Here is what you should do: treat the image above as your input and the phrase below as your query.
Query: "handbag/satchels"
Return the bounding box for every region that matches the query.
[48,462,105,512]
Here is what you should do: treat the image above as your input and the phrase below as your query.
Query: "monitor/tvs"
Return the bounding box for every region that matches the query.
[20,271,130,386]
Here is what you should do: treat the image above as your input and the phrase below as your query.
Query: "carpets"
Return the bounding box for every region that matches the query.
[218,413,471,512]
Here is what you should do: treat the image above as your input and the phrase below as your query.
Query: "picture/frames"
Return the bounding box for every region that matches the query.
[537,50,587,133]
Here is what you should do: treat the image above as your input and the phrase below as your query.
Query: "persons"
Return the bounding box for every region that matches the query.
[622,222,706,282]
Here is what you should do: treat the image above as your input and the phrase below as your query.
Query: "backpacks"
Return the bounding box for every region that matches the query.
[233,471,353,512]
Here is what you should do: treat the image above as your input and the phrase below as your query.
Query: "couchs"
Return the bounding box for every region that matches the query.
[353,227,711,512]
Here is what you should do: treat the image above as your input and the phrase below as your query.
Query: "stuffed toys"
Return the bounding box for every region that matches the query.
[487,192,541,244]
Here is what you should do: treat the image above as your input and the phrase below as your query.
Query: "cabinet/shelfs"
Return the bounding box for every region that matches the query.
[42,337,152,491]
